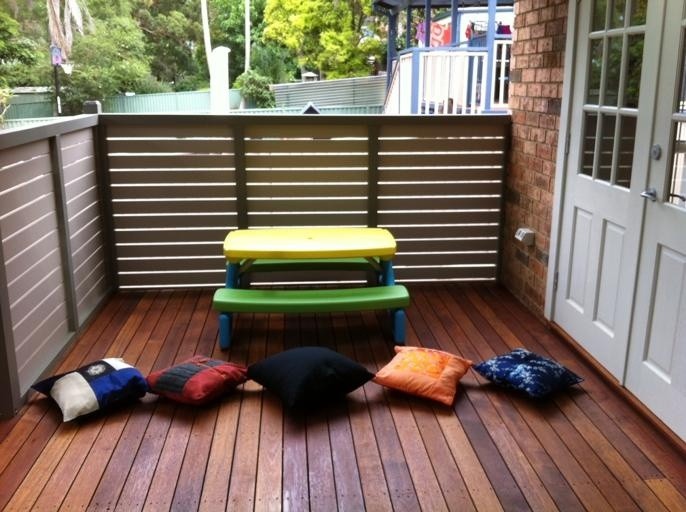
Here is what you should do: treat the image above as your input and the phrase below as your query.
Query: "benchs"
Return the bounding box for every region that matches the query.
[211,226,419,347]
